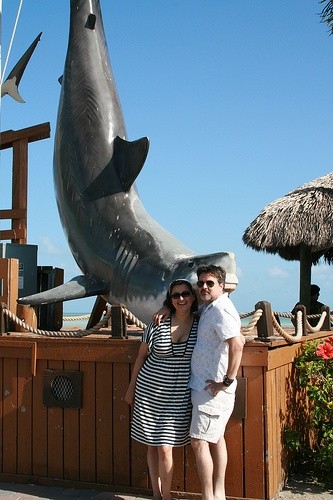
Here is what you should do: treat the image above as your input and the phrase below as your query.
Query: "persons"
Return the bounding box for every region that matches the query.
[153,265,244,500]
[291,285,325,327]
[125,278,200,500]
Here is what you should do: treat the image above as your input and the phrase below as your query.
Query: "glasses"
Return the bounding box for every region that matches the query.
[196,280,214,288]
[171,290,192,299]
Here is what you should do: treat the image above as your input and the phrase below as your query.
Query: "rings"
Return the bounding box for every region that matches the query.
[211,388,214,390]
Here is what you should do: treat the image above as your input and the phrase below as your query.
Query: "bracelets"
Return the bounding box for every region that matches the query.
[223,375,234,388]
